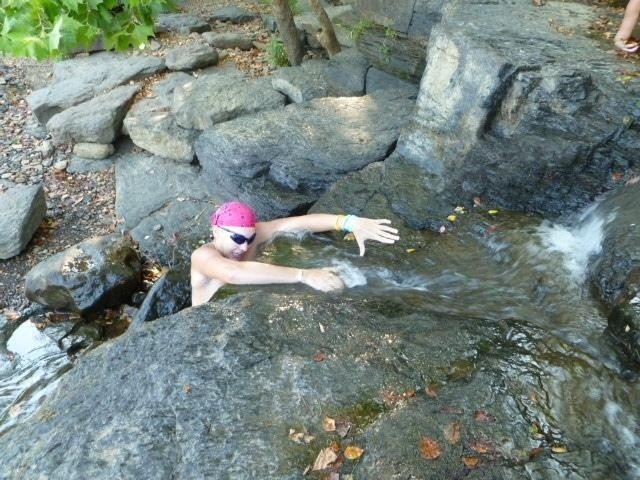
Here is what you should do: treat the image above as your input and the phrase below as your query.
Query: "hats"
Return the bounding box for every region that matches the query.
[210,202,257,227]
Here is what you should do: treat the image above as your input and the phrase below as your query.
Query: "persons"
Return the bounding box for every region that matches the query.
[189,200,401,321]
[614,0,640,54]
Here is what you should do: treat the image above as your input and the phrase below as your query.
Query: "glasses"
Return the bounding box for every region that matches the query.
[218,226,256,245]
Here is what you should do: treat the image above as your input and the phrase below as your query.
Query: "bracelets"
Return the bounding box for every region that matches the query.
[336,215,342,231]
[298,269,303,282]
[341,215,351,230]
